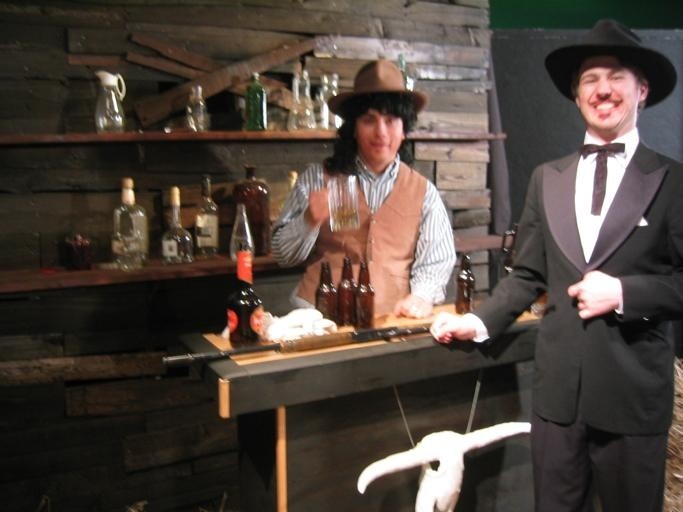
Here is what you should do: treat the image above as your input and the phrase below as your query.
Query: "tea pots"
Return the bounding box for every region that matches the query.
[93,70,127,133]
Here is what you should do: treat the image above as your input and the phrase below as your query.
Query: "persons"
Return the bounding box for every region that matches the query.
[271,60,457,323]
[429,19,683,511]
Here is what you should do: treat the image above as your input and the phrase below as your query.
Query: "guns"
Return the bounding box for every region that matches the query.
[160,320,434,365]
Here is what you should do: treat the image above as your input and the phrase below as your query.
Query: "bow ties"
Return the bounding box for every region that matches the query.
[580,143,627,160]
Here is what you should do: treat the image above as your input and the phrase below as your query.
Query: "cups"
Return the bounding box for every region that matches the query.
[328,173,359,231]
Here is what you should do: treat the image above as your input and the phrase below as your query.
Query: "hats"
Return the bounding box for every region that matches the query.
[544,18,676,110]
[328,60,430,118]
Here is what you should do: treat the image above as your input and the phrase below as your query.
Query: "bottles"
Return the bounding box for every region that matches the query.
[315,74,331,129]
[286,69,301,130]
[338,256,357,325]
[399,54,418,130]
[277,170,299,211]
[112,164,271,269]
[315,261,338,321]
[246,71,267,130]
[228,249,263,348]
[299,70,316,130]
[185,85,197,131]
[455,251,476,316]
[332,71,345,128]
[356,260,373,329]
[194,85,206,131]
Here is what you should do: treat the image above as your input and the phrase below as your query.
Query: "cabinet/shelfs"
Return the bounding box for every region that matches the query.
[169,299,543,512]
[0,128,515,298]
